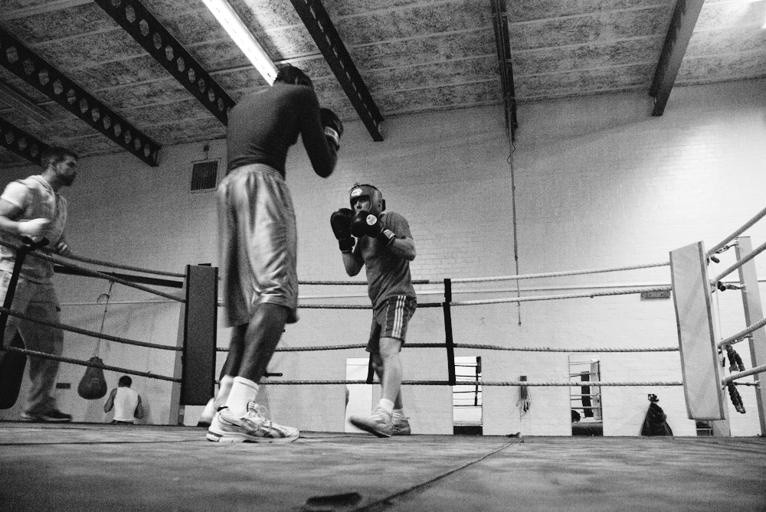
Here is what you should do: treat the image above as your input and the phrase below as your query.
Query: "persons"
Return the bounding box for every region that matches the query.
[1,145,78,420]
[197,66,344,444]
[330,185,418,437]
[104,375,144,425]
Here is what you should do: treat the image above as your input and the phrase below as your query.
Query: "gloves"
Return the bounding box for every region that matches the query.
[330,209,355,253]
[320,107,345,152]
[352,210,396,246]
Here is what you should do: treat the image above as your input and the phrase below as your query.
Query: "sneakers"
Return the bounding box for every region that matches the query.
[207,400,300,444]
[21,408,72,422]
[350,406,393,438]
[391,418,411,436]
